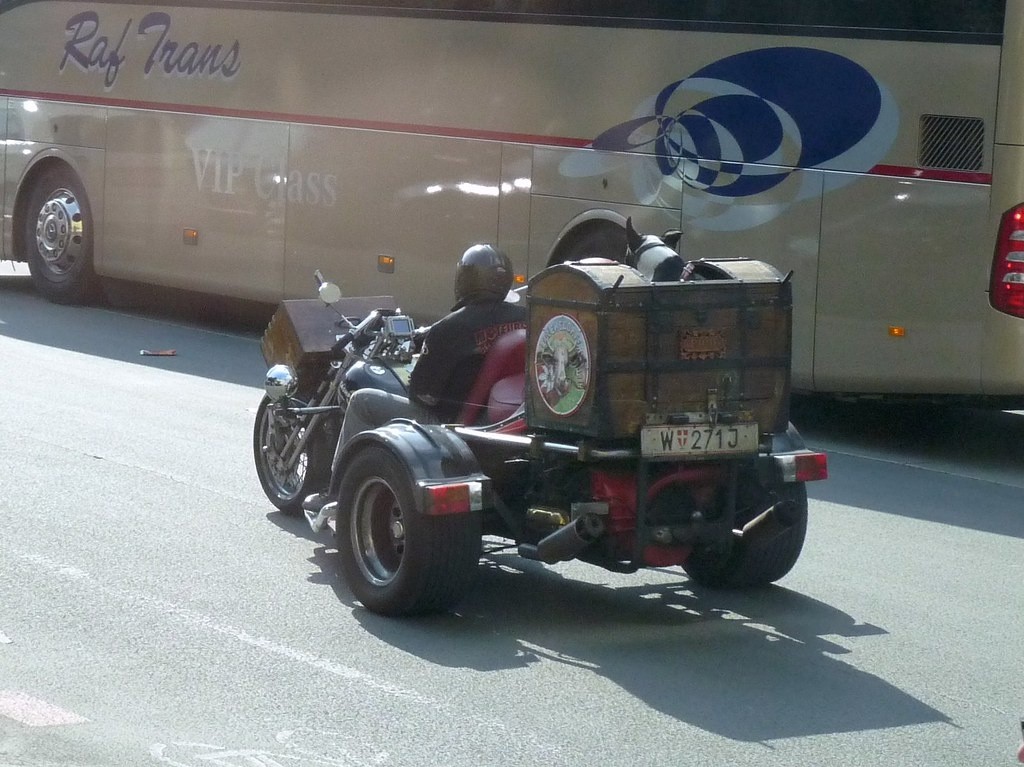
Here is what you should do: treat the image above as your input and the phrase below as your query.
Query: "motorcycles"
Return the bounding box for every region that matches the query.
[253,269,830,616]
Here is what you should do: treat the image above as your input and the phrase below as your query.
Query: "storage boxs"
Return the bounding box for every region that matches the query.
[523,255,794,441]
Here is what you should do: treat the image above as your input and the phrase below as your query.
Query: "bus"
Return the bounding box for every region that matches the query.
[0,0,1023,403]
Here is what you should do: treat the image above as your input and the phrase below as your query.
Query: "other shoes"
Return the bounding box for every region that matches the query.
[303,493,339,512]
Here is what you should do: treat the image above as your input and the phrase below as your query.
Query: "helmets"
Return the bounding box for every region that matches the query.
[454,244,514,300]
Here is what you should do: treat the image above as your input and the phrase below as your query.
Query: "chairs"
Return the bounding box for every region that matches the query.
[456,326,526,427]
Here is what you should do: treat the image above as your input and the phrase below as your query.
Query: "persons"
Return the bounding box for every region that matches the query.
[301,242,527,515]
